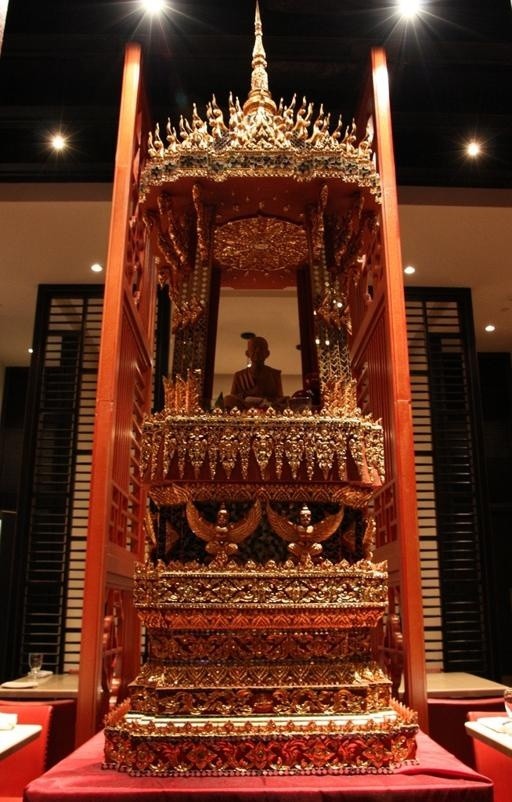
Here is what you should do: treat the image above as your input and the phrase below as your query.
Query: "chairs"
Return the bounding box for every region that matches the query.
[427,697,511,801]
[1,699,81,802]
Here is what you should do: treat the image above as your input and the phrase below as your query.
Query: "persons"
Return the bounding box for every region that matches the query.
[223,336,283,412]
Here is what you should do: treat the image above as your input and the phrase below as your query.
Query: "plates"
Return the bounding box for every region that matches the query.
[2,681,38,689]
[29,671,53,677]
[479,716,509,733]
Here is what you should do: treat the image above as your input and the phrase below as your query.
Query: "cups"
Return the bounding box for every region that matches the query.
[503,691,512,717]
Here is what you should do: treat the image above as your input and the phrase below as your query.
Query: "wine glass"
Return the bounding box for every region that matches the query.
[29,653,43,681]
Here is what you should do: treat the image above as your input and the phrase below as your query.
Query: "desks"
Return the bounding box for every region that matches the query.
[25,727,492,802]
[0,671,116,700]
[399,670,512,704]
